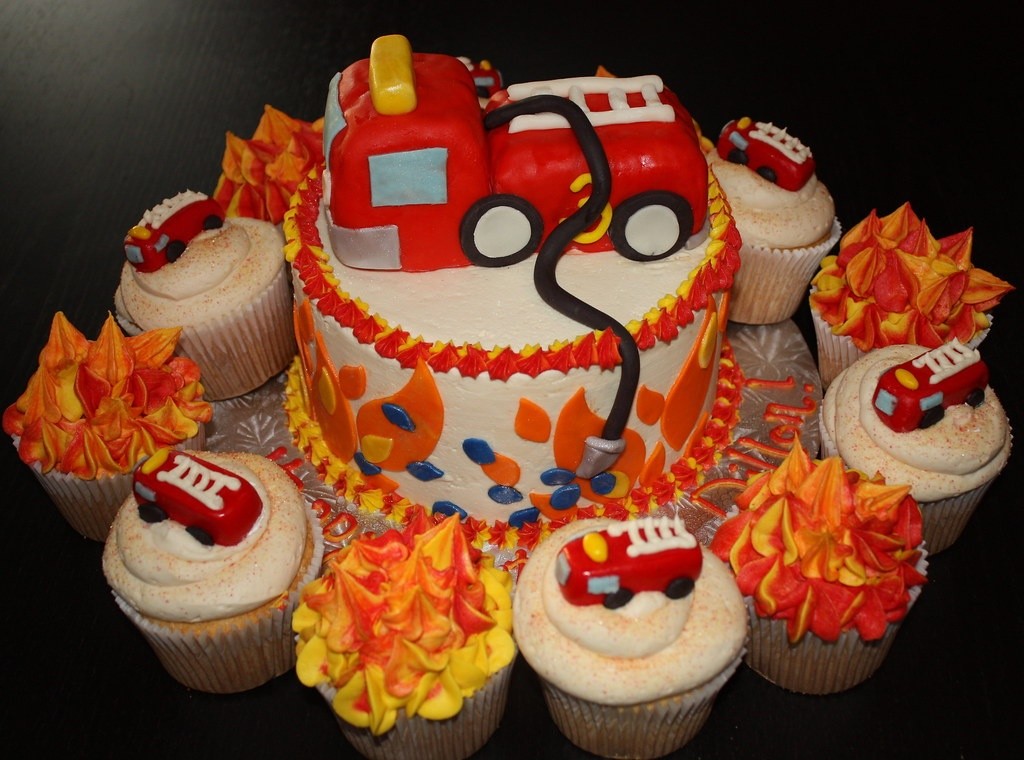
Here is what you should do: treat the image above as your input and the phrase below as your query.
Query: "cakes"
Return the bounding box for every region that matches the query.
[283,33,740,549]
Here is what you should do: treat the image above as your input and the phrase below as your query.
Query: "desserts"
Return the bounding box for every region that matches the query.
[6,102,1018,760]
